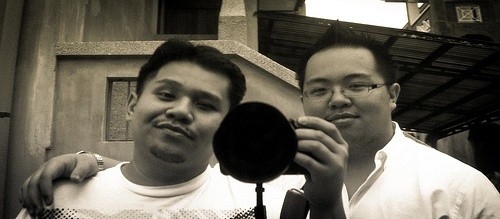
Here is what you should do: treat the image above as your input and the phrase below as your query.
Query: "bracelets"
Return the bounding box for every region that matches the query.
[76,150,106,171]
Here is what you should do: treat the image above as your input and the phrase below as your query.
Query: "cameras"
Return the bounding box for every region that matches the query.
[212,102,315,184]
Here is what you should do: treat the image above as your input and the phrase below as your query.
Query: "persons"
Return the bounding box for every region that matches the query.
[15,37,351,219]
[17,20,500,219]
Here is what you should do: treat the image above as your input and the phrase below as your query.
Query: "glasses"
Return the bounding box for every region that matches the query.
[301,82,389,101]
